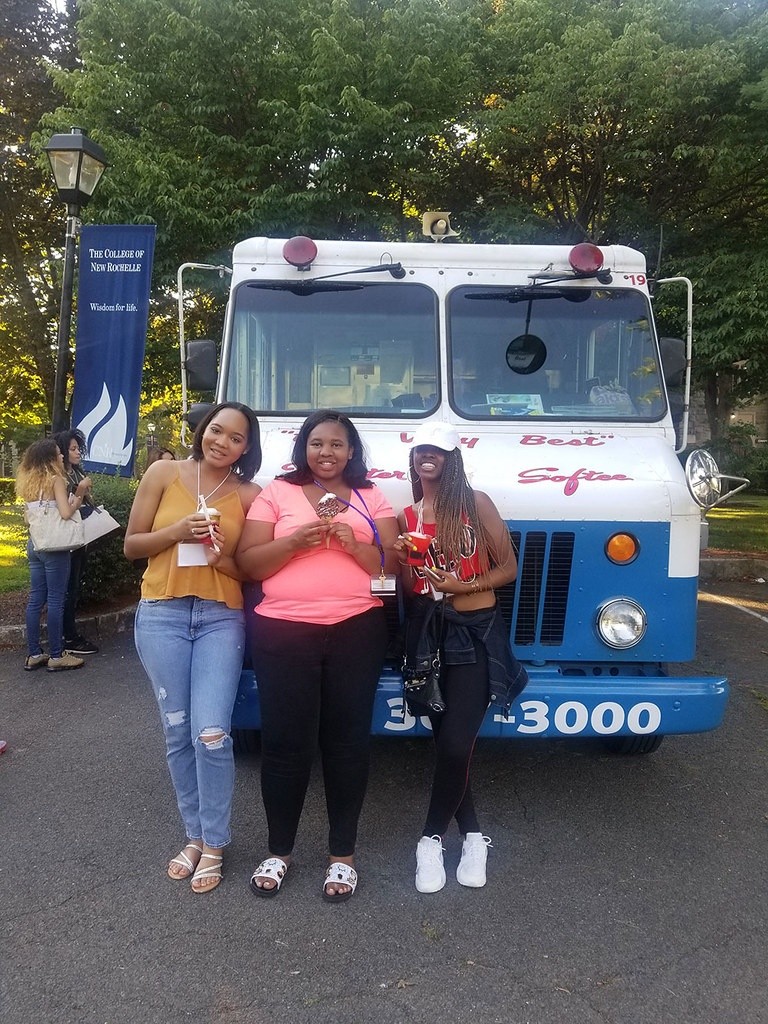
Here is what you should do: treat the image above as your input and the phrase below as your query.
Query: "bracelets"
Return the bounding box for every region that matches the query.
[466,579,481,598]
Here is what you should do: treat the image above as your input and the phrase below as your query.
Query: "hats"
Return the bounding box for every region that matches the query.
[410,421,462,452]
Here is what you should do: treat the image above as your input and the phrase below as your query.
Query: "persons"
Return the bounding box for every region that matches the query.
[147,448,176,464]
[235,411,401,901]
[16,442,93,671]
[54,432,99,653]
[393,422,517,893]
[125,402,262,893]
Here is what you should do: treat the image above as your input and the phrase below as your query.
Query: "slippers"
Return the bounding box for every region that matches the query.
[250,858,291,896]
[323,862,358,902]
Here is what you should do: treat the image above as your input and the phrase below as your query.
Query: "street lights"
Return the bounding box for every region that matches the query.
[40,124,109,444]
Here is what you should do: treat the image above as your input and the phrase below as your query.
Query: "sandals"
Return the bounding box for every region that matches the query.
[168,843,203,880]
[190,853,224,894]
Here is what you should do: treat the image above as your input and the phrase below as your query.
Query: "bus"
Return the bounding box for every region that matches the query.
[177,234,730,756]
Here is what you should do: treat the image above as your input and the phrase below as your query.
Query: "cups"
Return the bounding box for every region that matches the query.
[407,533,432,567]
[199,513,221,542]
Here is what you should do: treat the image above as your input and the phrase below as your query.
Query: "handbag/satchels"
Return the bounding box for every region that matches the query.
[25,476,85,552]
[66,485,121,549]
[402,653,446,715]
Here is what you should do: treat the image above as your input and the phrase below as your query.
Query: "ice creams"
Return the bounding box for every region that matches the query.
[316,492,340,549]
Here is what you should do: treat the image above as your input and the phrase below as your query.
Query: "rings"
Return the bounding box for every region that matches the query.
[192,528,195,534]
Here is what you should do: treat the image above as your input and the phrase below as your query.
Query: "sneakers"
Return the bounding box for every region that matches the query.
[415,835,447,893]
[23,653,52,670]
[456,833,494,887]
[46,652,85,671]
[62,636,98,654]
